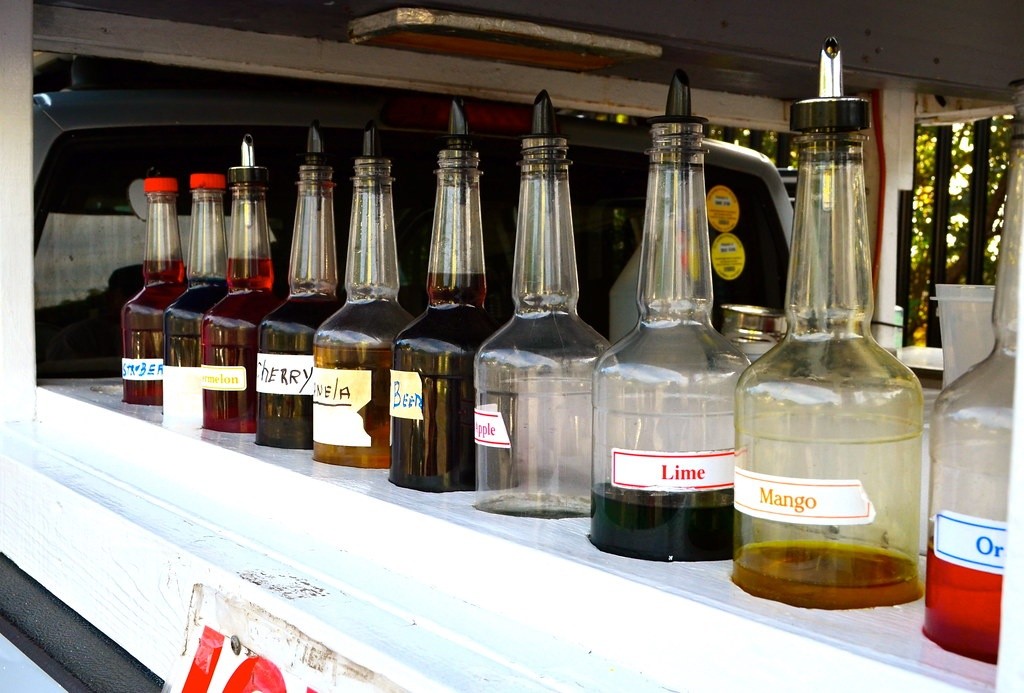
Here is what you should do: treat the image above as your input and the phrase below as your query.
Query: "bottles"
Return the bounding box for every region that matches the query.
[587,115,760,563]
[920,82,1023,672]
[163,173,233,425]
[253,153,345,449]
[470,135,620,521]
[202,166,285,433]
[310,158,416,469]
[731,98,946,610]
[388,133,499,494]
[120,177,189,404]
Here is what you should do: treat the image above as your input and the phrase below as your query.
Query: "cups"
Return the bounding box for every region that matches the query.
[719,302,789,364]
[930,282,997,392]
